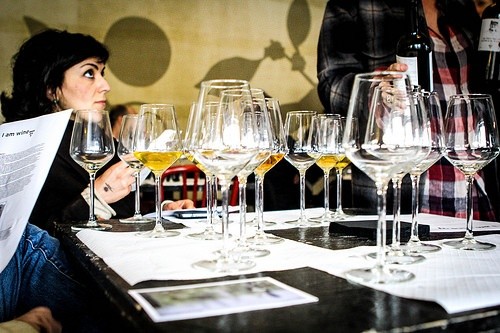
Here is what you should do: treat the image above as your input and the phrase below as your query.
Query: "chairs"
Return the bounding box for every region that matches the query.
[160,166,239,209]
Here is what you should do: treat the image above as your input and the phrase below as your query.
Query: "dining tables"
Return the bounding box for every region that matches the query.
[52,206,500,333]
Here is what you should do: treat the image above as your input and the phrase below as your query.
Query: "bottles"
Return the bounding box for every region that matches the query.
[395,0,435,123]
[466,0,500,99]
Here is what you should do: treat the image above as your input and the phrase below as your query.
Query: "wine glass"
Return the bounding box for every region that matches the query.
[69,109,115,231]
[383,91,446,254]
[279,110,322,227]
[324,117,359,218]
[133,104,183,238]
[307,114,346,222]
[438,93,499,252]
[364,84,431,266]
[116,114,155,224]
[342,70,420,285]
[181,78,289,276]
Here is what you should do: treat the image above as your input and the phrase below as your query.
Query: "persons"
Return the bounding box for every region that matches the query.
[0,30,194,223]
[317,0,500,221]
[0,223,89,333]
